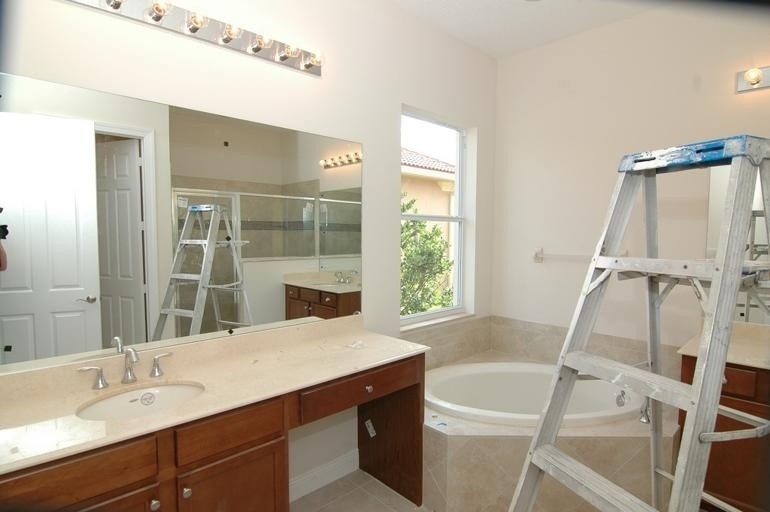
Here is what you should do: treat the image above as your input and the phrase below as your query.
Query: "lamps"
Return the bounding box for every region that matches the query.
[737,66,770,92]
[71,0,321,78]
[320,152,362,168]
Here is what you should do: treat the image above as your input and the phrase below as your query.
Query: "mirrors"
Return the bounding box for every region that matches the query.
[0,73,362,363]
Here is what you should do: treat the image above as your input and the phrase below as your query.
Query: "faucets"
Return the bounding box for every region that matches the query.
[110,336,123,353]
[120,348,138,383]
[334,271,345,283]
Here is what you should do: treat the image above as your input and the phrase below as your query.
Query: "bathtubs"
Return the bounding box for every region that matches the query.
[425,362,645,427]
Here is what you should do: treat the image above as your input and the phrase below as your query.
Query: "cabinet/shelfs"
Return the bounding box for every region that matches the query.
[285,285,361,320]
[0,348,426,512]
[678,356,770,512]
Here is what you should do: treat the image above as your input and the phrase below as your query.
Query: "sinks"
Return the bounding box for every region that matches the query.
[75,378,205,420]
[312,284,341,288]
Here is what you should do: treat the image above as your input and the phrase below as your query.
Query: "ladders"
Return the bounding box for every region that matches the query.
[151,204,254,342]
[510,134,770,512]
